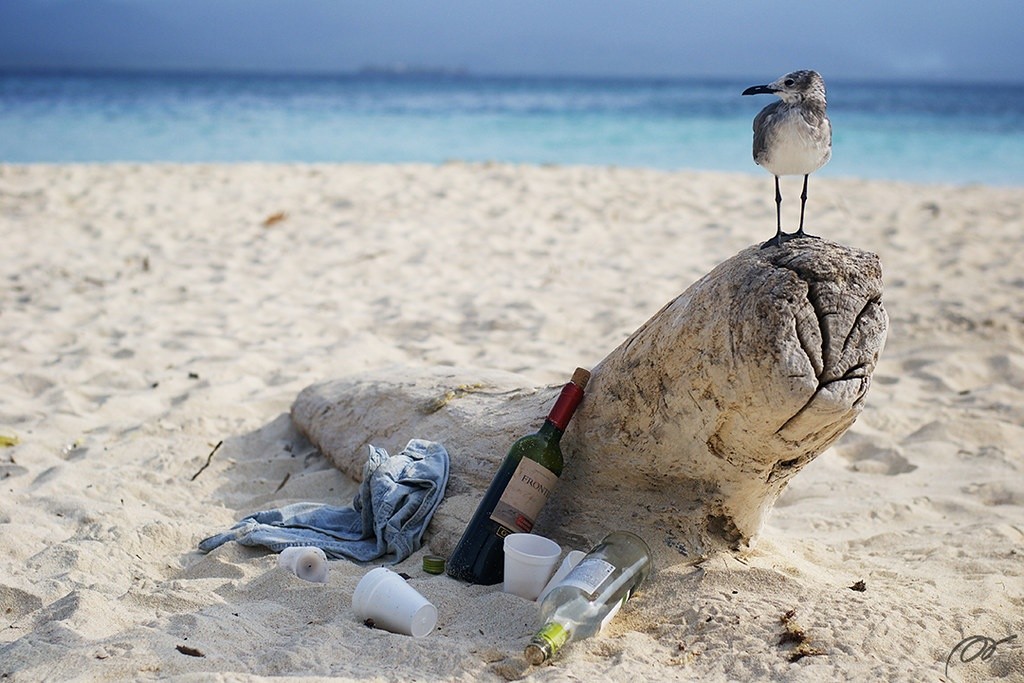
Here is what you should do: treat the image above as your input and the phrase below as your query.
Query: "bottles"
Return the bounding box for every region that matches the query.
[445,367,590,587]
[525,529,653,666]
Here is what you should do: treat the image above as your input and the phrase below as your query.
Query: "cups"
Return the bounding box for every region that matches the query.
[503,533,563,602]
[537,550,588,605]
[350,568,438,638]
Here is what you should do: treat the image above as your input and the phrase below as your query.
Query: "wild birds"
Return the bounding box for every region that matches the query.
[743,69,832,248]
